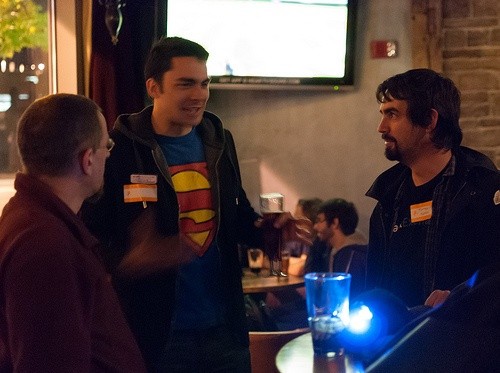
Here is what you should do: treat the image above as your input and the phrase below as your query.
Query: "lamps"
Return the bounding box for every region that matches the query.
[100,0,126,46]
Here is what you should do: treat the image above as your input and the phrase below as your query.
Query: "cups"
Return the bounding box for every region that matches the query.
[270,249,289,278]
[304,272,351,358]
[247,248,264,274]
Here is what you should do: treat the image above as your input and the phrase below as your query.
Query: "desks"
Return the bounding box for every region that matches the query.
[275,331,366,373]
[242,267,306,293]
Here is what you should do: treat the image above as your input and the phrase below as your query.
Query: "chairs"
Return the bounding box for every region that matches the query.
[248,328,311,373]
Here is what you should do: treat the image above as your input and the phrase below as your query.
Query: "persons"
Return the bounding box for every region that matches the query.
[1,93,147,373]
[245,197,369,332]
[364,68,500,373]
[85,36,314,373]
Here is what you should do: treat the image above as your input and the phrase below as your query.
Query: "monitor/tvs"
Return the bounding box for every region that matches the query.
[154,0,357,93]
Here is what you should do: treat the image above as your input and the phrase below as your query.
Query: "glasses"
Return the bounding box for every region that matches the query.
[78,136,115,158]
[312,217,326,225]
